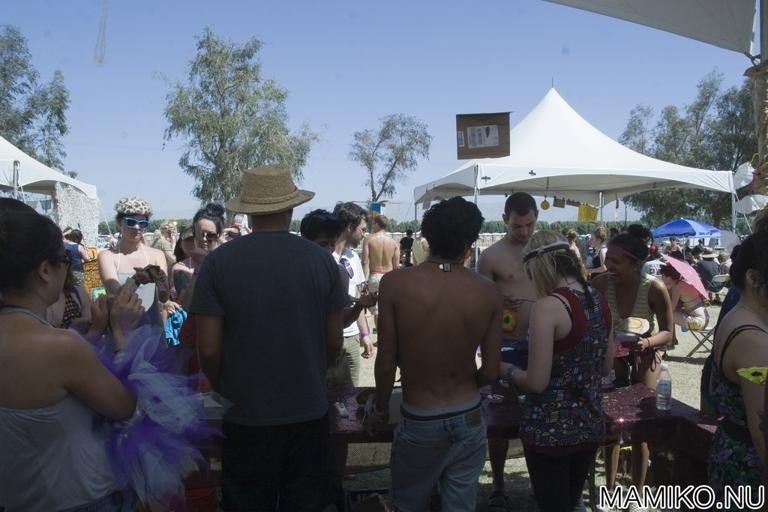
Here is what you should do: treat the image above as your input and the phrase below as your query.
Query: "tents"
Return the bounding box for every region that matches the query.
[413,87,737,232]
[0,136,97,246]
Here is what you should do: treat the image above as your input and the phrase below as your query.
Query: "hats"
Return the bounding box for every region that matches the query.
[223,164,316,215]
[179,224,193,241]
[113,197,152,217]
[699,248,719,258]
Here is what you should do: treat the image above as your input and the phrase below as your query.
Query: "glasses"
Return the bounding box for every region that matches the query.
[32,248,76,269]
[121,216,151,229]
[190,228,217,242]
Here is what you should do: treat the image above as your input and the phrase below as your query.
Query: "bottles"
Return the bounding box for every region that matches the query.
[657,363,671,412]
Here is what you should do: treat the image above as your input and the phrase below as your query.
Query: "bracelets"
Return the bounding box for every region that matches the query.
[647,337,651,348]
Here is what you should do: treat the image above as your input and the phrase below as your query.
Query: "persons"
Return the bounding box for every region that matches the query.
[664,236,682,261]
[564,228,585,275]
[171,204,224,313]
[46,250,92,338]
[61,227,94,291]
[375,197,502,511]
[494,229,614,512]
[684,237,721,257]
[468,245,481,269]
[692,246,723,306]
[0,197,137,512]
[221,221,250,241]
[584,226,608,280]
[410,234,432,265]
[709,253,734,305]
[188,161,352,512]
[623,225,661,279]
[479,191,542,512]
[332,200,375,392]
[657,263,709,351]
[301,208,380,330]
[362,214,400,348]
[151,218,179,289]
[174,225,194,265]
[694,237,705,255]
[97,197,170,331]
[693,223,768,512]
[589,224,676,512]
[398,227,415,268]
[684,248,697,272]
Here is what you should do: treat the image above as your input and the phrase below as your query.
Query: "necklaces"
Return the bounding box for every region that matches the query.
[424,258,465,272]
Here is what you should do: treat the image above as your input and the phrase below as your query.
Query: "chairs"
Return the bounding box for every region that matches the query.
[340,387,405,512]
[687,323,717,357]
[706,274,730,304]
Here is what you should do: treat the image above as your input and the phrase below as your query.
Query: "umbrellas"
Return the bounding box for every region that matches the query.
[661,254,709,300]
[649,217,720,236]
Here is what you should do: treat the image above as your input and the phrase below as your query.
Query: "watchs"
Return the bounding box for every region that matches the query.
[504,364,518,382]
[356,333,370,340]
